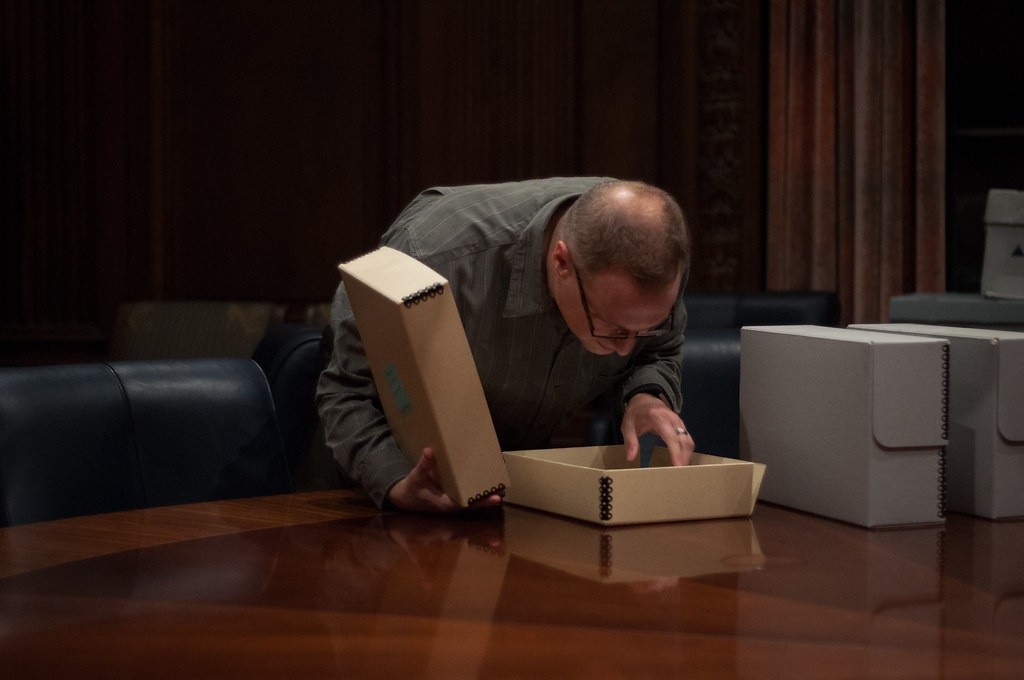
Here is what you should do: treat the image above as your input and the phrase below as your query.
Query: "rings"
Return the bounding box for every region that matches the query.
[675,427,688,435]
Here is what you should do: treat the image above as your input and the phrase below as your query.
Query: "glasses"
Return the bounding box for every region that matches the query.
[566,251,676,339]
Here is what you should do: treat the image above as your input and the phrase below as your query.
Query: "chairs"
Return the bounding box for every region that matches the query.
[0,320,331,528]
[670,292,847,464]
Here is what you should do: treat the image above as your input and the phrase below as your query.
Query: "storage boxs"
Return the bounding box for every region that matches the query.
[339,189,1024,528]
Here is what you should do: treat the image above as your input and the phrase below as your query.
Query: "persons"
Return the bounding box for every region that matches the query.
[314,176,696,516]
